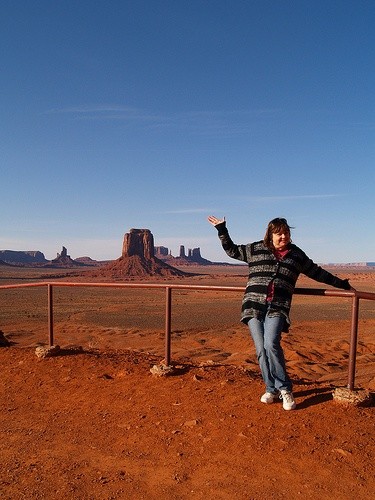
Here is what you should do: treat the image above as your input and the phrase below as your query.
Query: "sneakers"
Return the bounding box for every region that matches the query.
[280,390,297,411]
[261,391,281,404]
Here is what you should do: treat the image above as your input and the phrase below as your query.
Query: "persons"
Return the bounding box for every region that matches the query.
[207,214,356,411]
